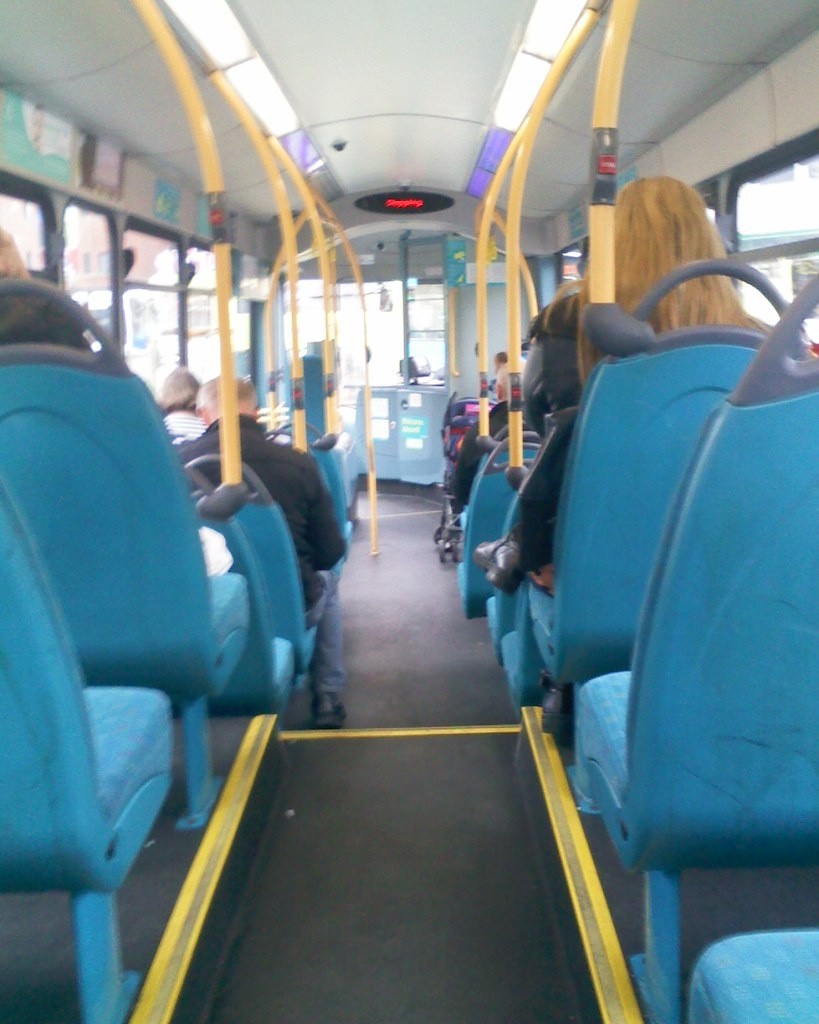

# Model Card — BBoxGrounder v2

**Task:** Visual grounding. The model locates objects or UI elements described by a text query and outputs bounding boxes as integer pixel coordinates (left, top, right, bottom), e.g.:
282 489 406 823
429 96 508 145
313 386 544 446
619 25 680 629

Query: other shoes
311 692 347 729
539 669 574 733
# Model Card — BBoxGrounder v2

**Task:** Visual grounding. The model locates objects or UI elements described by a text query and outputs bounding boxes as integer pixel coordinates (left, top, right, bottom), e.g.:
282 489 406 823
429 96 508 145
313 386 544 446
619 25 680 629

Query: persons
524 235 590 434
452 358 535 514
0 227 94 350
493 352 508 402
155 366 209 447
174 374 349 730
464 172 762 594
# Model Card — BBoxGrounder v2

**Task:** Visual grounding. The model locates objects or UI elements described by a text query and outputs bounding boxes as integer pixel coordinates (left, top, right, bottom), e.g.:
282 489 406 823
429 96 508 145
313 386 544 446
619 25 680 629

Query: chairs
182 452 318 673
278 422 354 564
177 465 296 716
0 477 174 1024
0 278 247 832
501 579 547 714
455 431 546 619
265 428 344 578
686 924 819 1024
295 356 328 437
527 259 809 811
460 419 540 541
583 267 819 1024
486 488 522 668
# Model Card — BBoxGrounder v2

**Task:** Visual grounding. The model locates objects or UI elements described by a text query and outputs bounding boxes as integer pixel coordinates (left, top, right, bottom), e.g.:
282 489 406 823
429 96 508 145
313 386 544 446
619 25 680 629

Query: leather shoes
473 533 527 594
